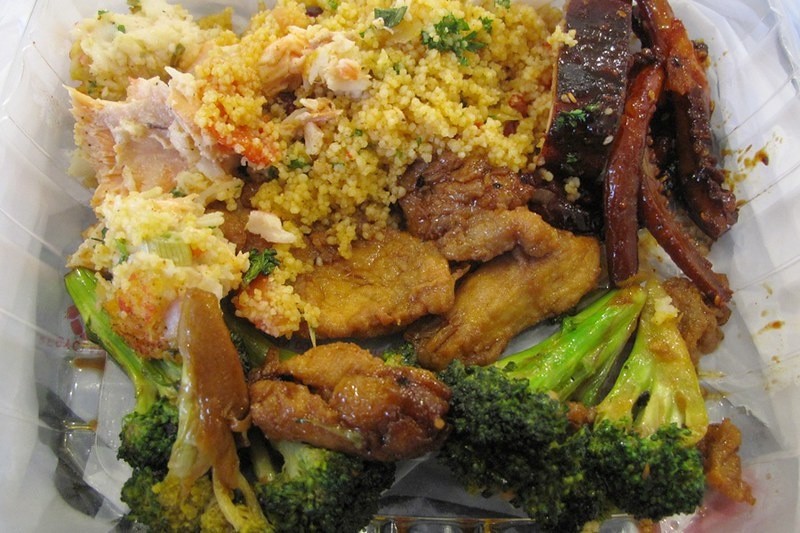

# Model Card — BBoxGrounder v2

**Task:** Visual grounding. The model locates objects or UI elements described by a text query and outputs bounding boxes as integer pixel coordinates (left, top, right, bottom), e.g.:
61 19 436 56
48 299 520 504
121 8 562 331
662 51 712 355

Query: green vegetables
374 6 599 128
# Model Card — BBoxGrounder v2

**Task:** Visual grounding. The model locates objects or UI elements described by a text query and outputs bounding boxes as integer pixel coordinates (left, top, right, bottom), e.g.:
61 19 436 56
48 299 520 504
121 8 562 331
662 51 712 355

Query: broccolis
65 249 708 533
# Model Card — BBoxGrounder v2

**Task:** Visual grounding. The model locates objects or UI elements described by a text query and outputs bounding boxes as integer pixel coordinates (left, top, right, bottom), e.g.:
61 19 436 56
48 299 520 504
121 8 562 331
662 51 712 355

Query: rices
68 0 579 342
